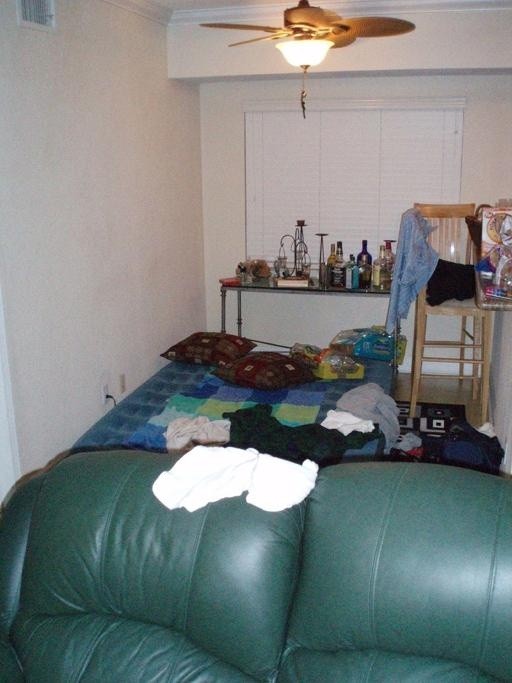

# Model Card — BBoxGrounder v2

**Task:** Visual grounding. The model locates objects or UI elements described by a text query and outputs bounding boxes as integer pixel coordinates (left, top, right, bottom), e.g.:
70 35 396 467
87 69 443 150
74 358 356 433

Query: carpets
375 401 466 462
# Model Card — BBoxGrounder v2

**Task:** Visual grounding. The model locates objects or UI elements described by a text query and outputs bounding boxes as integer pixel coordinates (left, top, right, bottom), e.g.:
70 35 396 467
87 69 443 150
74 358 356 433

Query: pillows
160 332 258 368
210 352 315 392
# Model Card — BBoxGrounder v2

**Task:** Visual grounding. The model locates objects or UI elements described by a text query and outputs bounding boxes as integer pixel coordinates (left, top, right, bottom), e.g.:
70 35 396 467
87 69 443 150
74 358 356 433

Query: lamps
274 39 335 120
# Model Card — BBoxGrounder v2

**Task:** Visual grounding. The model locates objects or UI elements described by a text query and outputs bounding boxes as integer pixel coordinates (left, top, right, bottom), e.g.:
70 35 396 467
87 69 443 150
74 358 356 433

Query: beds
71 352 395 465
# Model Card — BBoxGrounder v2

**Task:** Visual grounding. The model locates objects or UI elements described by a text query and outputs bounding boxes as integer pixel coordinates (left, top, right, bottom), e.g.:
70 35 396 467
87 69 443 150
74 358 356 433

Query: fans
198 1 415 48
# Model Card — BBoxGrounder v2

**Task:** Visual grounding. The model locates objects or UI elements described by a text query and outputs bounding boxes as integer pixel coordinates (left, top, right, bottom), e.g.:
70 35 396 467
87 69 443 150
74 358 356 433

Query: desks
219 276 416 374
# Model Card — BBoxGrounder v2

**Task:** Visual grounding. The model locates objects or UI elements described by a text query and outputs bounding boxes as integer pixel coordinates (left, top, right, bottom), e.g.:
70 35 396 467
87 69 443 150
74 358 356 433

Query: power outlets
101 384 109 405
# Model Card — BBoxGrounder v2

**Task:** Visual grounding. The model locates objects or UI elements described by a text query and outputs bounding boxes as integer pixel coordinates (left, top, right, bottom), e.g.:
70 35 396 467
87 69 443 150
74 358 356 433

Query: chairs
408 202 492 426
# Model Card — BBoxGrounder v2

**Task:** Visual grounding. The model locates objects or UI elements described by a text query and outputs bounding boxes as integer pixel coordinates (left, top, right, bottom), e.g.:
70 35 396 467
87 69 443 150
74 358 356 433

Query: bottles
343 254 353 268
346 256 359 289
331 241 346 287
359 254 372 288
378 257 394 292
385 240 395 264
357 240 372 266
327 243 336 281
372 245 385 289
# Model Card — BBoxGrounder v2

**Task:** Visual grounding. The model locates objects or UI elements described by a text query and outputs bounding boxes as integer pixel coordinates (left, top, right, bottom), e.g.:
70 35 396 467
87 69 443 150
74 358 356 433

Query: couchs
276 461 511 683
0 448 309 683
